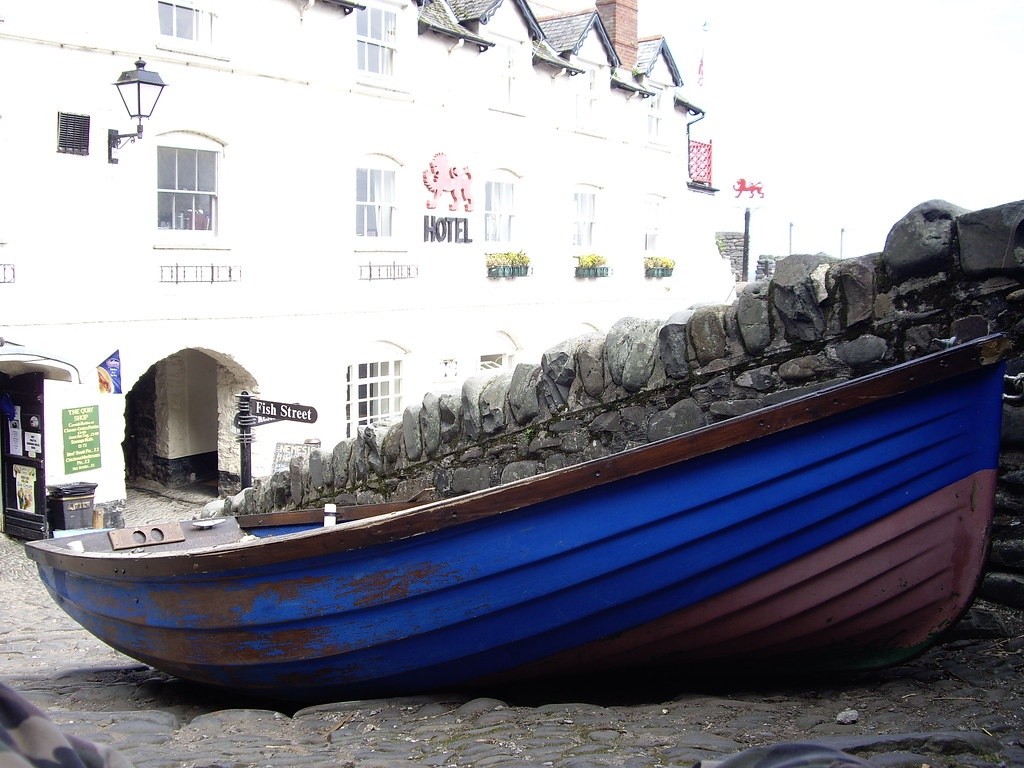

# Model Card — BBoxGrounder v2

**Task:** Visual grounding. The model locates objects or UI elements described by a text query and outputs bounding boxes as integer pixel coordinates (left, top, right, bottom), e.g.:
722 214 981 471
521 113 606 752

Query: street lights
840 228 845 257
789 222 793 255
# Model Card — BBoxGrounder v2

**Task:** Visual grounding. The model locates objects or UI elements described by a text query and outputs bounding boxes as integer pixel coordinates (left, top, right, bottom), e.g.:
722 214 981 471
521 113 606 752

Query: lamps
108 57 170 163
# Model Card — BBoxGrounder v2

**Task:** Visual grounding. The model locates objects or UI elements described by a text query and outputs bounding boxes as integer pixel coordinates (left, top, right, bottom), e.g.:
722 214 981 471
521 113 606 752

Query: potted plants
644 256 675 277
576 255 610 278
487 252 531 279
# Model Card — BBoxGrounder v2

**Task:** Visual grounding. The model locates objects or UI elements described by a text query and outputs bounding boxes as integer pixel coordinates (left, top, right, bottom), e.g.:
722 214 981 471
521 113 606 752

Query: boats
24 329 1013 716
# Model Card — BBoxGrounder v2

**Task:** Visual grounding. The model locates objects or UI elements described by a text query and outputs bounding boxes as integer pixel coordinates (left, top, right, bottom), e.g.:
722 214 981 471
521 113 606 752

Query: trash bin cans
47 481 98 528
